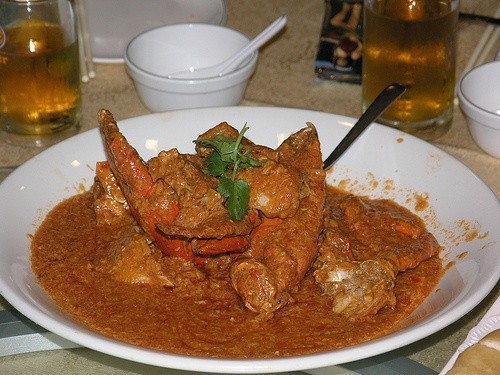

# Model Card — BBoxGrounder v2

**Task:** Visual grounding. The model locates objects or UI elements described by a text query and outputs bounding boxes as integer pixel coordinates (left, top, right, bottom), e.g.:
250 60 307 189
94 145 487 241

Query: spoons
167 16 288 78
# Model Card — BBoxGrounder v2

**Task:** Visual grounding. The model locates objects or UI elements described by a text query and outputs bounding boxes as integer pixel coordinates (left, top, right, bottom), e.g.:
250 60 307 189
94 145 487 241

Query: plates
94 0 227 64
0 106 500 374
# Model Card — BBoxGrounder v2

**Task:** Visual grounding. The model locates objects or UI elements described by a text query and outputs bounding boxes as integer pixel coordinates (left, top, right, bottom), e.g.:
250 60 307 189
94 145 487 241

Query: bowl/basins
457 60 500 159
124 22 258 113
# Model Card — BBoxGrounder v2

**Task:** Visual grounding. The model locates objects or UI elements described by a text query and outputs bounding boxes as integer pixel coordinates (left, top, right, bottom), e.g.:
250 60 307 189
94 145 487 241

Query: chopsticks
75 0 96 83
463 7 500 82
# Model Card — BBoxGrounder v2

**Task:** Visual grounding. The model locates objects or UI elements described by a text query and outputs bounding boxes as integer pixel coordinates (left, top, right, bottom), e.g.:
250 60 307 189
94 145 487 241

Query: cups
0 0 82 148
362 0 459 141
